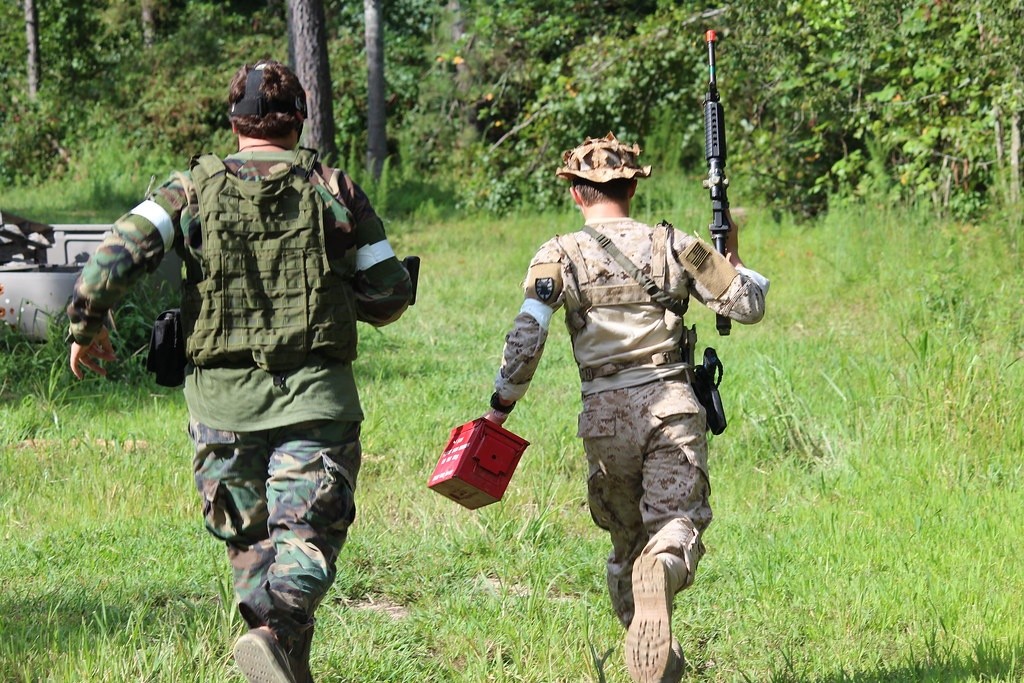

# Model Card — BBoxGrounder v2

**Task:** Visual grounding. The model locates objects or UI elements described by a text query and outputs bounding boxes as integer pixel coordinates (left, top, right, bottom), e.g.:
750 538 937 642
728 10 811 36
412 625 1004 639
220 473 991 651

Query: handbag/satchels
146 308 184 387
690 365 727 435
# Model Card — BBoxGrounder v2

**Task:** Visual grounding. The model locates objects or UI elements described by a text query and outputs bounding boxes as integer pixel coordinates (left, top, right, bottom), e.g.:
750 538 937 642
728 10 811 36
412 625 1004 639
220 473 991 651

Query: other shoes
625 553 684 683
233 629 314 683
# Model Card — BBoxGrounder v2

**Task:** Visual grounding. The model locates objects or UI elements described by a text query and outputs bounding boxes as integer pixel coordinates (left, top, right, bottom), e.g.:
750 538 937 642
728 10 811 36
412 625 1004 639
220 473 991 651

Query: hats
555 131 651 183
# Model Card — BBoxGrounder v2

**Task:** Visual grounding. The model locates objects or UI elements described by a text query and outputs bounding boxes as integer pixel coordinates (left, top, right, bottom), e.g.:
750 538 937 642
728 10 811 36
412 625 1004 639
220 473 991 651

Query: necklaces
239 144 289 152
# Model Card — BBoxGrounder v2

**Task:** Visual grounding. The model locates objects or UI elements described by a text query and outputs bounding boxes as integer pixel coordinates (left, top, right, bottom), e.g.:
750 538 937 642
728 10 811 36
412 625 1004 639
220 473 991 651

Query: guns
703 29 733 337
698 345 724 404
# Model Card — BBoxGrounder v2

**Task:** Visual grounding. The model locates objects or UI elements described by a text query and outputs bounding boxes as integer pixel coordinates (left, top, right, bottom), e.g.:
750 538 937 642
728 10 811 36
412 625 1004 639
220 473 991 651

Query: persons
65 60 420 683
484 130 770 683
